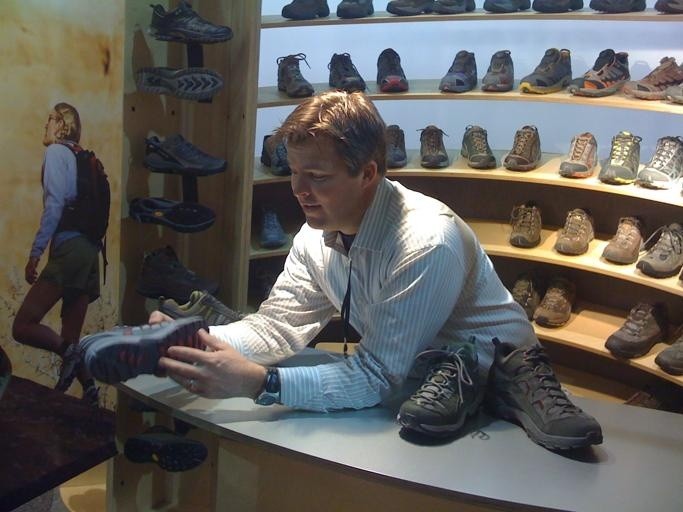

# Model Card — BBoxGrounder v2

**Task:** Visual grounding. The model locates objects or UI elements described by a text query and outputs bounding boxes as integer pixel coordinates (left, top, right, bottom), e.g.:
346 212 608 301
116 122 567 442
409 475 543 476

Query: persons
148 89 546 413
10 102 111 407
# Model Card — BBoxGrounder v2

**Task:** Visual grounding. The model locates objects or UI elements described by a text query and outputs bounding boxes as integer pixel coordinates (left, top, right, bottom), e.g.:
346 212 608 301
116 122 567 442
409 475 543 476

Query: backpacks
57 141 110 237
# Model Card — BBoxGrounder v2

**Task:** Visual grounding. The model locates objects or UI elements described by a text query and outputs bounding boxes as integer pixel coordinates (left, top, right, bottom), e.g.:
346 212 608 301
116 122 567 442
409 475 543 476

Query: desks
0 375 118 512
112 347 683 512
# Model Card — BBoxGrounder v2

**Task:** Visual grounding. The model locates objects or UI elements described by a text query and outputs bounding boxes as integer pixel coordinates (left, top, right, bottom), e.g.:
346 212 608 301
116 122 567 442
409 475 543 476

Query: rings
188 380 197 392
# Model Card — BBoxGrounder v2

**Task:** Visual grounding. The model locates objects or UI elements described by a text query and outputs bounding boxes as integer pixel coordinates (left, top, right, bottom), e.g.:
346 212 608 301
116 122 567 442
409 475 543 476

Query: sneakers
55 343 98 403
486 337 603 450
143 134 228 178
397 336 487 437
276 48 408 99
260 134 291 176
135 243 247 325
77 316 210 384
258 201 286 248
136 66 224 99
505 125 540 172
128 196 216 232
384 123 450 169
558 132 683 188
605 300 683 411
460 126 497 168
124 425 207 472
510 200 683 281
149 3 234 44
439 49 683 105
510 271 576 328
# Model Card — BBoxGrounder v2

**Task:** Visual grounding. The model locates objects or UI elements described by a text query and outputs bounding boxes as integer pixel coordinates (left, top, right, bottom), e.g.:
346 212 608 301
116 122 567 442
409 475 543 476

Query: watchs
254 367 281 406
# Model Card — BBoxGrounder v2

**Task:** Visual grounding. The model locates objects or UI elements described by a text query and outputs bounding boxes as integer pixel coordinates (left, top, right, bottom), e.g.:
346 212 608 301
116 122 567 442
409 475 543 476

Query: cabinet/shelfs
119 0 683 415
